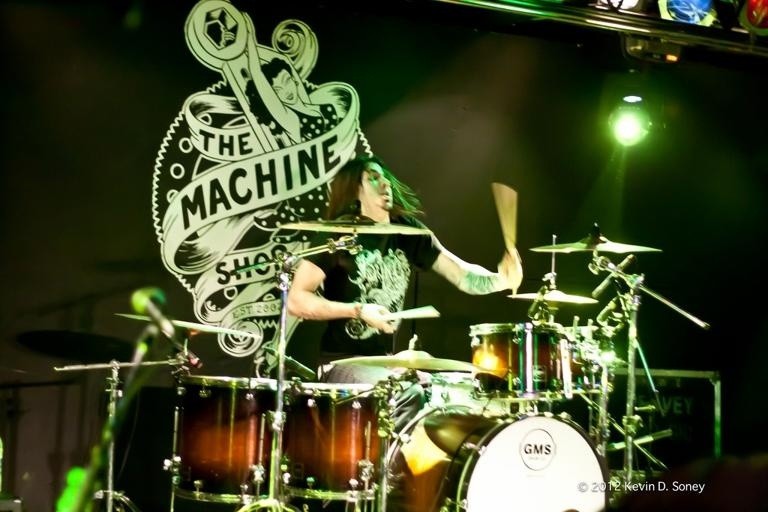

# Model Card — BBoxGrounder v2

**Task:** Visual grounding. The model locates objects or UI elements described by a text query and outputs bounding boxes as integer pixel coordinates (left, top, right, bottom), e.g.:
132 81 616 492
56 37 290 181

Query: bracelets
355 297 363 320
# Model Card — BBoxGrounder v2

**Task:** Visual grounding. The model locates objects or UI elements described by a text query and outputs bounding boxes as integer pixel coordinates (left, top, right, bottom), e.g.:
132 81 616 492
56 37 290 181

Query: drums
281 383 387 503
175 373 289 508
468 323 562 386
384 401 608 512
560 325 606 378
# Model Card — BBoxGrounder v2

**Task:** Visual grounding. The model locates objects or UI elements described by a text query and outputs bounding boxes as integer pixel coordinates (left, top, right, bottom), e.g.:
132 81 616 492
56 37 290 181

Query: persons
285 158 523 432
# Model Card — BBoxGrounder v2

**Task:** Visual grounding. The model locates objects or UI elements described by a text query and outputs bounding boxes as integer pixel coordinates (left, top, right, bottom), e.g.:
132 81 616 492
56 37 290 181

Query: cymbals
507 291 600 306
123 311 261 339
529 238 664 255
280 215 431 240
329 350 473 373
